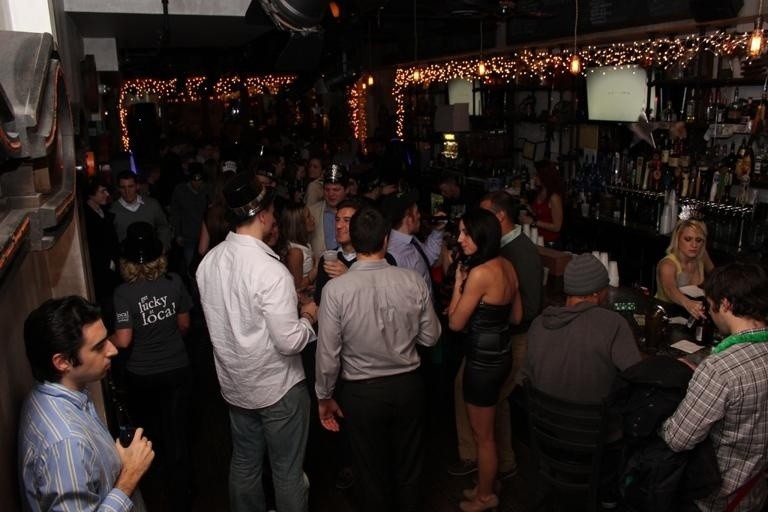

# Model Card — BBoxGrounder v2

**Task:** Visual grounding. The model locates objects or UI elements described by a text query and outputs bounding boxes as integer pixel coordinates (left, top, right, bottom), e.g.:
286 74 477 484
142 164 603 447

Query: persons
651 218 716 323
662 261 766 511
83 142 567 510
15 296 157 511
510 250 662 511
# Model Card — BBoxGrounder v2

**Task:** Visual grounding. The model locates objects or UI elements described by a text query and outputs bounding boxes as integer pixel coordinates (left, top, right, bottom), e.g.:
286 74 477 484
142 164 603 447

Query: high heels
459 495 498 512
463 483 498 500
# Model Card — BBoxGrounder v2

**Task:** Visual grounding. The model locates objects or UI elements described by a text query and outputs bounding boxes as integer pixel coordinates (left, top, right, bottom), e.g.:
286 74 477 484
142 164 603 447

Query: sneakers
496 458 516 481
447 458 479 476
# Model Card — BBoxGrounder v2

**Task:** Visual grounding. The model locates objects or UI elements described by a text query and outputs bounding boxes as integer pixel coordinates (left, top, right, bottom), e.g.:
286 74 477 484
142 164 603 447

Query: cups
580 202 588 217
322 250 338 264
514 222 619 290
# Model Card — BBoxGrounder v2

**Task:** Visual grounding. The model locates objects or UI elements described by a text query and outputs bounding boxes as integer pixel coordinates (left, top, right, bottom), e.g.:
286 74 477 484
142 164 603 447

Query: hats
319 163 348 186
221 170 275 223
114 219 164 265
562 254 610 295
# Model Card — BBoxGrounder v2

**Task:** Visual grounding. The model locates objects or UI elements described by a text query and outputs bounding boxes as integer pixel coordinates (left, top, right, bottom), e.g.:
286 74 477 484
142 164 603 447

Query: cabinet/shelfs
471 48 764 215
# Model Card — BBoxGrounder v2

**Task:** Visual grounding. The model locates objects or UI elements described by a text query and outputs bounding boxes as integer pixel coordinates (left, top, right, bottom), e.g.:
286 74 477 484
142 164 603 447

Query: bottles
113 399 136 448
609 84 768 208
492 168 529 191
583 154 597 175
695 307 714 346
